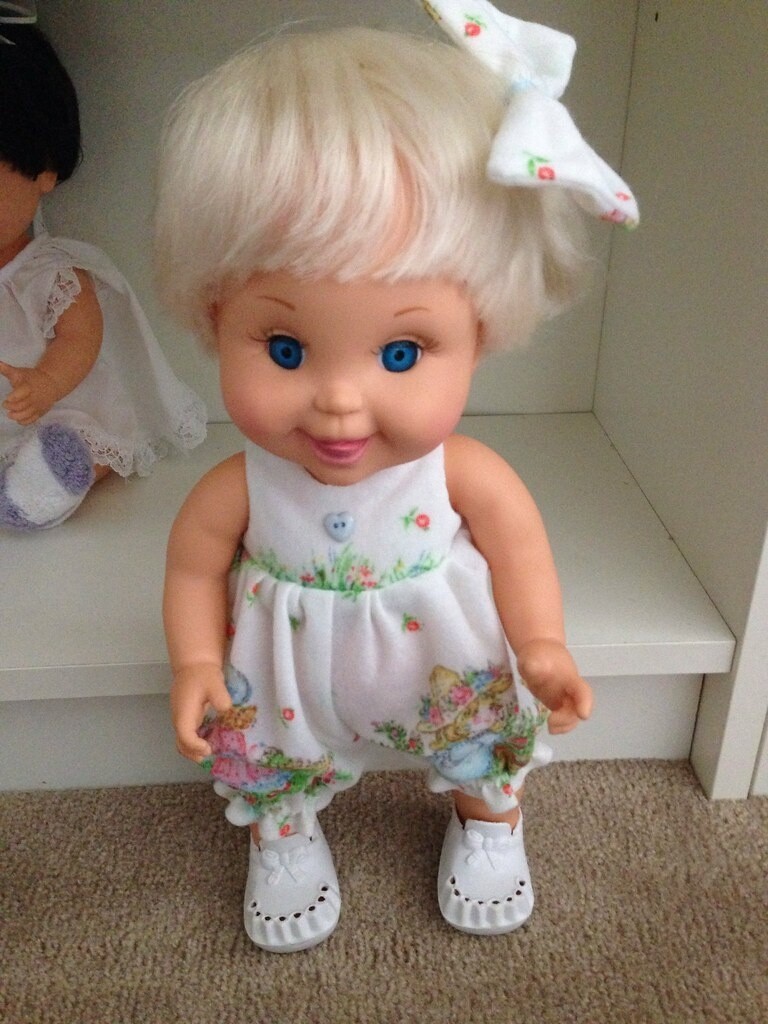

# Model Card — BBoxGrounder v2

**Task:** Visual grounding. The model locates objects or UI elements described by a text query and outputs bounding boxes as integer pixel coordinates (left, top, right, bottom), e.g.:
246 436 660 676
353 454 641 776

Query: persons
158 3 645 955
0 0 211 532
